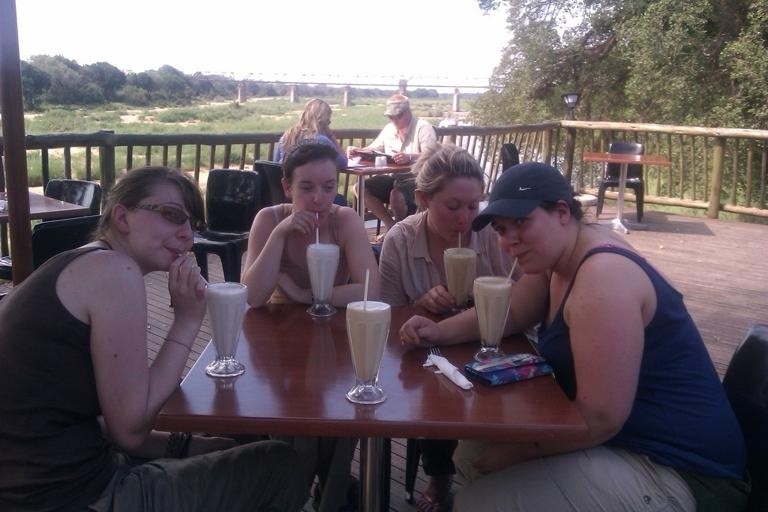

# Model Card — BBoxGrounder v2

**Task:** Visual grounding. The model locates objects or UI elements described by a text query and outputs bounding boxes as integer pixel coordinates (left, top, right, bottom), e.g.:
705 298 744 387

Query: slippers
415 490 448 512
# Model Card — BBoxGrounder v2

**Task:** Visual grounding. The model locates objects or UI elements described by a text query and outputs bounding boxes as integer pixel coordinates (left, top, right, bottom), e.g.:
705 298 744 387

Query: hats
470 162 576 233
383 94 410 116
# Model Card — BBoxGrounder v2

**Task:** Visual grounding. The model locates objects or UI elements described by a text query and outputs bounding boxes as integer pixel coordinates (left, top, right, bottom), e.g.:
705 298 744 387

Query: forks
430 345 442 360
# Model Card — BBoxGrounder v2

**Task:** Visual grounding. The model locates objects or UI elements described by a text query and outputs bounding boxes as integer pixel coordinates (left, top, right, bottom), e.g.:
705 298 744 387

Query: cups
375 156 387 168
306 244 340 317
204 281 248 378
473 276 513 363
345 301 392 404
443 248 476 312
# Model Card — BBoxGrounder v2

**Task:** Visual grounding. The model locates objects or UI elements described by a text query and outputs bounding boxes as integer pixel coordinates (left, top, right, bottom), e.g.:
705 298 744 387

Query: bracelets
327 131 335 138
166 431 192 459
408 156 412 163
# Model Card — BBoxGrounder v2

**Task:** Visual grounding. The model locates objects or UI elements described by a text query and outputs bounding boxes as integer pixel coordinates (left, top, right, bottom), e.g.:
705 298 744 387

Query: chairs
43 179 102 223
251 158 289 210
1 213 105 280
166 167 269 307
501 142 519 173
720 323 768 510
595 131 647 223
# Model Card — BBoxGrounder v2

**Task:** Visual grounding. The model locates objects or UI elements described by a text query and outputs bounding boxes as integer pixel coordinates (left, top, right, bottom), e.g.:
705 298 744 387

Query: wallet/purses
463 353 553 386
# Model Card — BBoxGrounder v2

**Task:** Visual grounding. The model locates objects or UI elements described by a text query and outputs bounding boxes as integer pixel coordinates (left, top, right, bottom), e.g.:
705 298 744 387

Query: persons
0 165 310 511
379 143 522 512
241 140 382 512
274 99 349 207
346 94 438 245
398 161 753 512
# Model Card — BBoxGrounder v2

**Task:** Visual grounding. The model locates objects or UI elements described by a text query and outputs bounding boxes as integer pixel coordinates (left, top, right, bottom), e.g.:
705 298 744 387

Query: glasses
128 202 209 237
